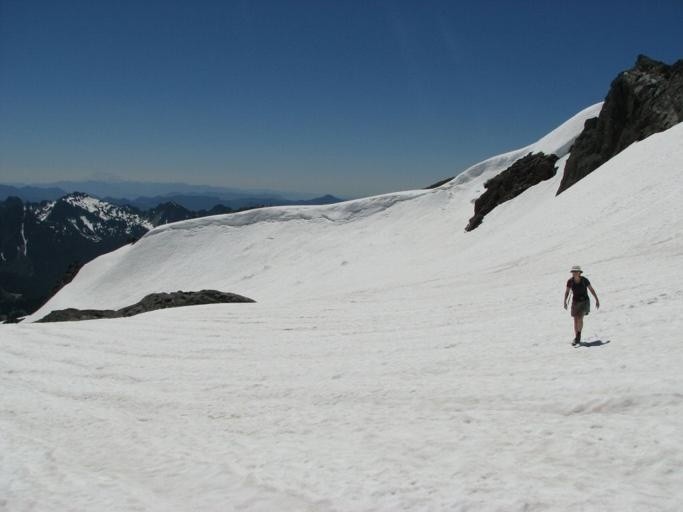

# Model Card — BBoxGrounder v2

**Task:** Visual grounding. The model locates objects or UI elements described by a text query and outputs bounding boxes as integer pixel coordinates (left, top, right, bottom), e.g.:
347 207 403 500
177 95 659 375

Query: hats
570 266 583 273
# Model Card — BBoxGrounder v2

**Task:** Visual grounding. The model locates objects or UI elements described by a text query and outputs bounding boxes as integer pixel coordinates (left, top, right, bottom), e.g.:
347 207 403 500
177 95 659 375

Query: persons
562 265 599 346
